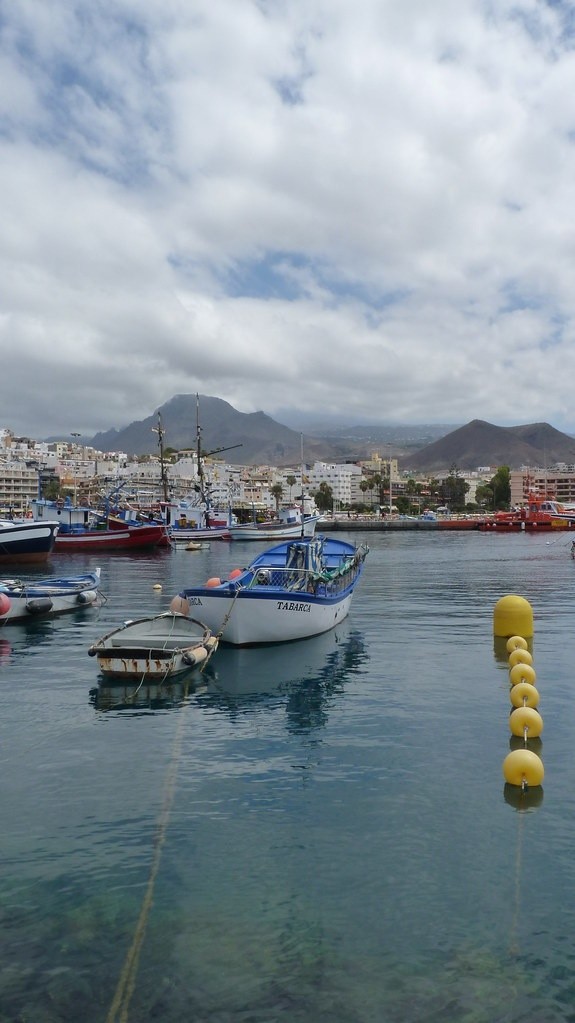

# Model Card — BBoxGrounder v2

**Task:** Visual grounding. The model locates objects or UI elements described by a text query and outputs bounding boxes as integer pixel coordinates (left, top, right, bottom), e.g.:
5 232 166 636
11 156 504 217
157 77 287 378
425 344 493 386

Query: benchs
112 635 204 649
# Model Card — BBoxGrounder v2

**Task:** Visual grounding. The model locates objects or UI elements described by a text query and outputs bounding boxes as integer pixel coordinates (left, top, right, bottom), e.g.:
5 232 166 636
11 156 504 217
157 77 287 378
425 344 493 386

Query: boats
1 599 101 661
87 610 211 679
88 669 209 717
0 516 60 565
0 568 101 624
181 434 371 648
476 498 575 531
11 470 170 554
90 481 323 544
